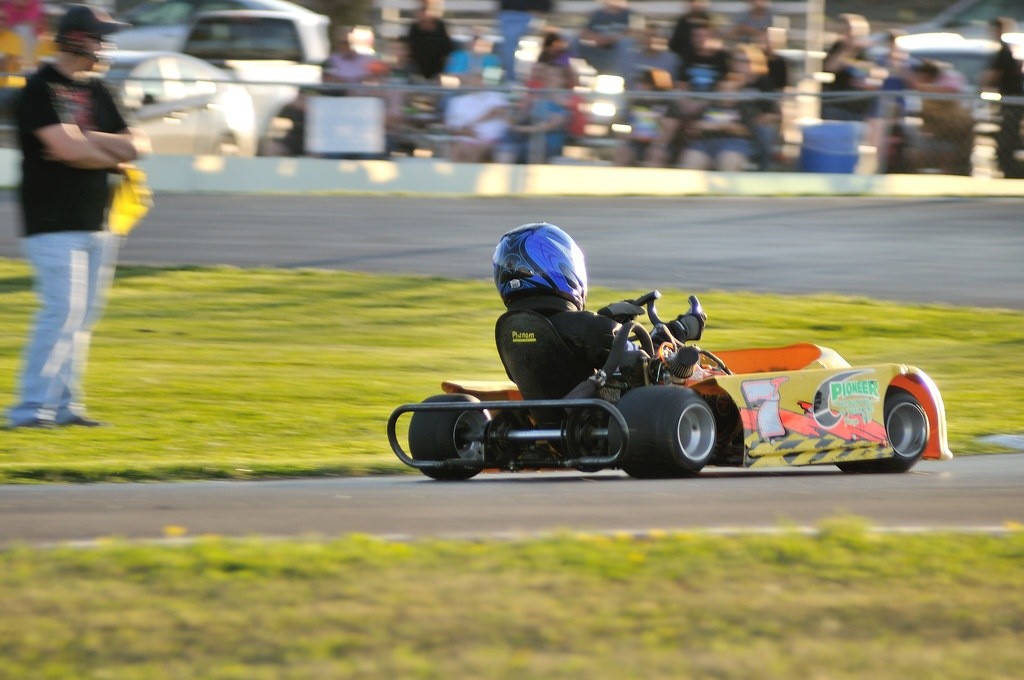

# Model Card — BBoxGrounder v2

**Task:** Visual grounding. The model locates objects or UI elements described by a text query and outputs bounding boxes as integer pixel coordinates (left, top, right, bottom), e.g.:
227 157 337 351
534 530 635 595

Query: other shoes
72 415 107 428
30 419 56 430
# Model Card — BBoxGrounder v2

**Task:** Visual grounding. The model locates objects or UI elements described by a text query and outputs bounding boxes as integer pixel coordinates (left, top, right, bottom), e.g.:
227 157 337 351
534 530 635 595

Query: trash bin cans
799 121 862 174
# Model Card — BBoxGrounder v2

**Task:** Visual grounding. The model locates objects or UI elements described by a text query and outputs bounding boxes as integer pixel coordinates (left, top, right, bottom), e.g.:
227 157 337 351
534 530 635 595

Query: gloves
667 313 707 342
599 299 646 322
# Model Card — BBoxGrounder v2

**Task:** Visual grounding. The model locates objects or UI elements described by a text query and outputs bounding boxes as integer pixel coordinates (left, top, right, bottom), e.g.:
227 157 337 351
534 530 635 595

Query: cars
99 1 331 67
0 50 258 160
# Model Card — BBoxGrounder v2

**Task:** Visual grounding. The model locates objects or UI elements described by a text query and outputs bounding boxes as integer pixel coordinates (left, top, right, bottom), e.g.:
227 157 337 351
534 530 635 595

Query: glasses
85 33 114 42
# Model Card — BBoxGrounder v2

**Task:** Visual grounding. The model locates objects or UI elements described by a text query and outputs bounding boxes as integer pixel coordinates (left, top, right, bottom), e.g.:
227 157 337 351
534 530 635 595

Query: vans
181 5 321 158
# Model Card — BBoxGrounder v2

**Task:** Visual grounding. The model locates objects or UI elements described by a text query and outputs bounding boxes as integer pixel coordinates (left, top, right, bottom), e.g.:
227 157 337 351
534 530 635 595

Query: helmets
493 222 587 310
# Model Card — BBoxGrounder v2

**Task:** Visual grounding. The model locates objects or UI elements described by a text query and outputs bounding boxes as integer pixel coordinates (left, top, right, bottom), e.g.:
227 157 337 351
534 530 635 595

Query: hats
58 4 132 34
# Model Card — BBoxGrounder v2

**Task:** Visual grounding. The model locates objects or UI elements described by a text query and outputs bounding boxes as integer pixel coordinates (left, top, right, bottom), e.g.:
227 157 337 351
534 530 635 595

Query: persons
492 220 704 372
272 0 1024 179
0 7 152 428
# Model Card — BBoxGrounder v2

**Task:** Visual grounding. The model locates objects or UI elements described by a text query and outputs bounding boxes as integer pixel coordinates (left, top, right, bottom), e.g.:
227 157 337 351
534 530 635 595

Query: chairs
495 308 631 425
